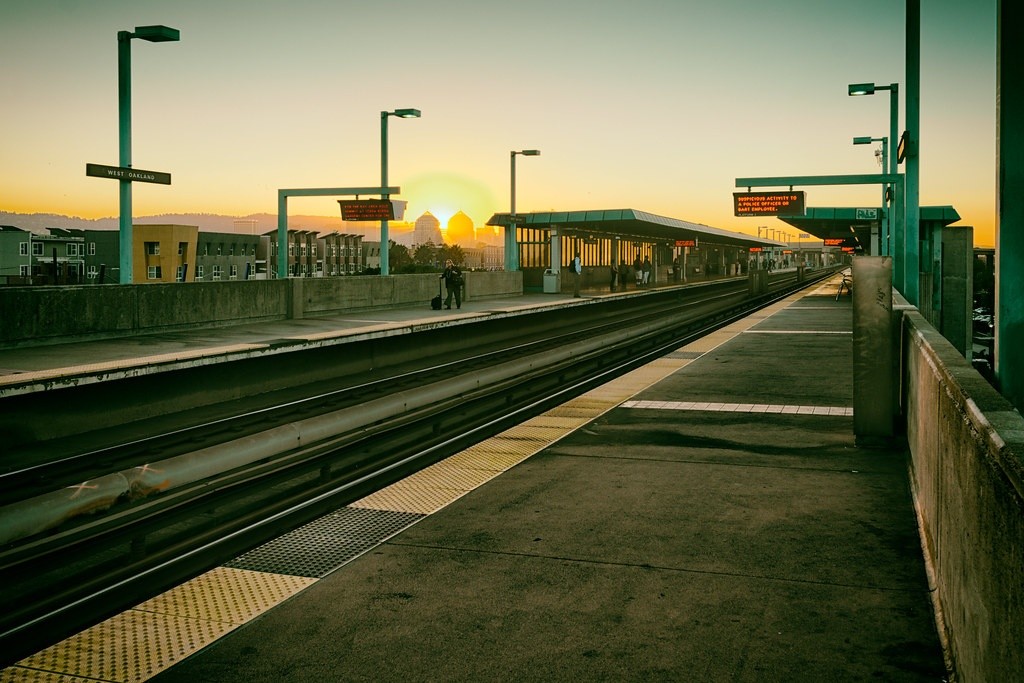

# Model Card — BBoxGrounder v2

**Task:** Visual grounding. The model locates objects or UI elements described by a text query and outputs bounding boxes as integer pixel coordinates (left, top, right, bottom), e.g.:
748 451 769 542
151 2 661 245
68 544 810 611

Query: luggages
431 278 442 310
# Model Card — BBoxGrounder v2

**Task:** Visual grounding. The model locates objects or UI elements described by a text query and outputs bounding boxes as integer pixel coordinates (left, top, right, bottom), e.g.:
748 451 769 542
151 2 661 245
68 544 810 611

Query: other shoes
574 295 580 298
457 306 460 309
447 306 451 309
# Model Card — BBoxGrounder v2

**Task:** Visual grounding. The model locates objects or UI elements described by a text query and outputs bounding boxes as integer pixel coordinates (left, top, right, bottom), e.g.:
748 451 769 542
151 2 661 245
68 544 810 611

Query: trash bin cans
542 268 563 294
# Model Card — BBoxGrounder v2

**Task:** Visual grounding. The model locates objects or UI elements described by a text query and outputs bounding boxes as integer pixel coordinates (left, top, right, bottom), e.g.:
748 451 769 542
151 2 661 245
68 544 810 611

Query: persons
439 260 462 310
644 256 651 282
618 259 629 291
734 255 788 276
641 260 650 285
573 253 582 297
703 260 710 280
633 255 643 287
671 258 678 280
610 258 618 292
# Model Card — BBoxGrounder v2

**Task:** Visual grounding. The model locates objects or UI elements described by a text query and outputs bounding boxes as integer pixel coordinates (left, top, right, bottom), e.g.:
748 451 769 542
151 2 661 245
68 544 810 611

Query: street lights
853 135 888 257
848 81 899 288
510 149 540 270
380 108 421 274
117 23 180 285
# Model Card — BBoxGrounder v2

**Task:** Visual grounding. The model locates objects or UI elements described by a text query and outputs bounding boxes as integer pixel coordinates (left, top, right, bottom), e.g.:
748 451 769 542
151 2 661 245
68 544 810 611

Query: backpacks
569 258 577 272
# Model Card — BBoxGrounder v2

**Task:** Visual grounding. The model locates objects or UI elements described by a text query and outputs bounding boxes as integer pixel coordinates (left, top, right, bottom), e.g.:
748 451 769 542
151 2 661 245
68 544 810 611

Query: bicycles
835 271 852 302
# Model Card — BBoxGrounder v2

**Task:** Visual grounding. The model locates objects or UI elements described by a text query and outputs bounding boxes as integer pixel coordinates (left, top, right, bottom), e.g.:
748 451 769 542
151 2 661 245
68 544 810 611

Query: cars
972 300 996 379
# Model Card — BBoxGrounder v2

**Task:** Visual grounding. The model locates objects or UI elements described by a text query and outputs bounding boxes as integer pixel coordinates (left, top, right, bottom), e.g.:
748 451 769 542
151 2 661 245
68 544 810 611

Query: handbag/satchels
455 277 464 285
